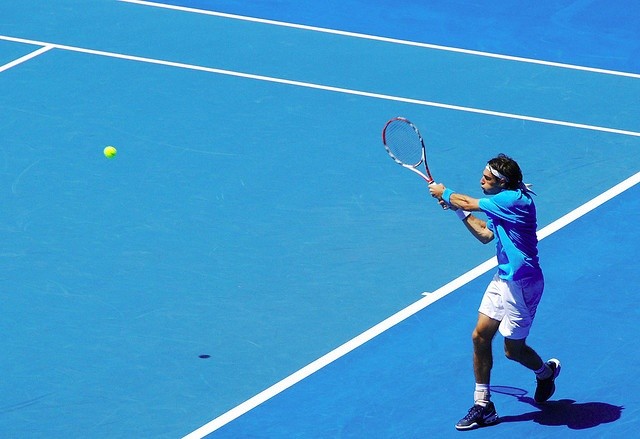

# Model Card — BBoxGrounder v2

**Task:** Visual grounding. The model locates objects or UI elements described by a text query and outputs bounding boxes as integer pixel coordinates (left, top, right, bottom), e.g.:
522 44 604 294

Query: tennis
103 146 118 160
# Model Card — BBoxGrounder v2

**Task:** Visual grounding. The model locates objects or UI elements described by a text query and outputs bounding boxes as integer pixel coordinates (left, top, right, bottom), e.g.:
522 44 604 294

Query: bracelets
455 208 472 220
441 188 454 204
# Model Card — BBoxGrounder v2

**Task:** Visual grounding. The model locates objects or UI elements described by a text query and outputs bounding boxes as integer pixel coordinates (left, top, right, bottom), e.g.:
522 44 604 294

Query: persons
428 153 561 430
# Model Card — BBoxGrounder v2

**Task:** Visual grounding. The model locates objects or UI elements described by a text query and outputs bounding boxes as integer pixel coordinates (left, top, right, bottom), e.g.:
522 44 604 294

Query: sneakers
533 357 561 404
454 402 499 430
